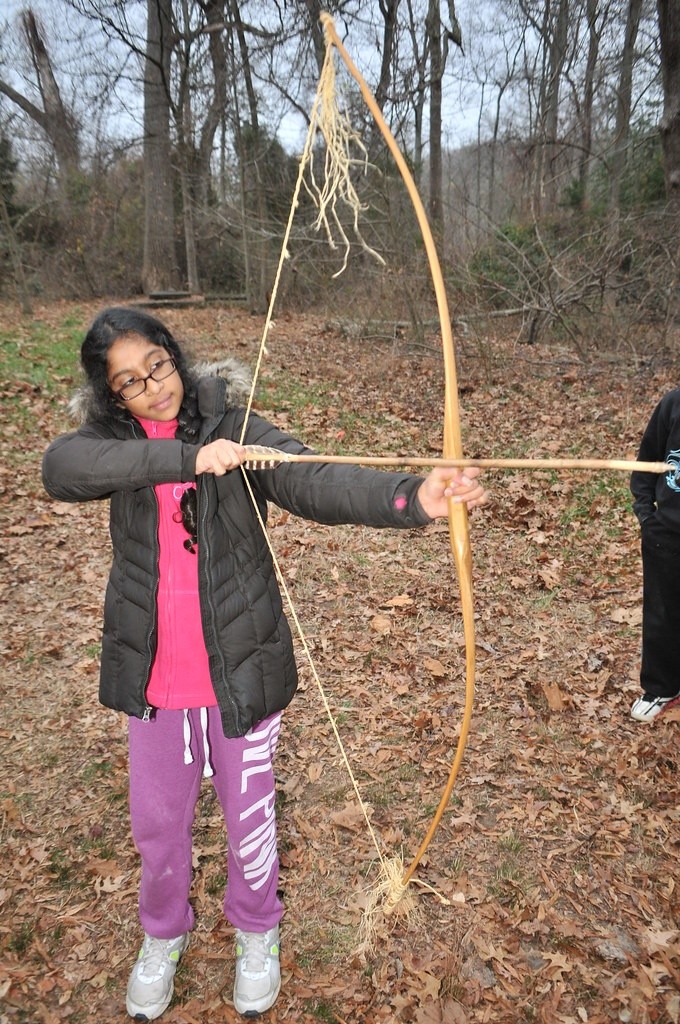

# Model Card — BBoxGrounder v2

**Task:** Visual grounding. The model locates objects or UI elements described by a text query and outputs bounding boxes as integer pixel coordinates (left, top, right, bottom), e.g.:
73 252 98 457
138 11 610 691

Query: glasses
109 344 178 402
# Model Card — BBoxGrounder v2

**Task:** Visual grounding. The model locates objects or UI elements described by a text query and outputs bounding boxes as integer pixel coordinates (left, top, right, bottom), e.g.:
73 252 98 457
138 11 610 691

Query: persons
41 307 490 1020
629 390 680 722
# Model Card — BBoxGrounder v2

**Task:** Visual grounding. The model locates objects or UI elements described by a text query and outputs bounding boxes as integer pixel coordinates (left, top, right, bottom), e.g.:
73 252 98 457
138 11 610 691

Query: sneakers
126 931 190 1019
630 691 680 720
232 922 281 1016
